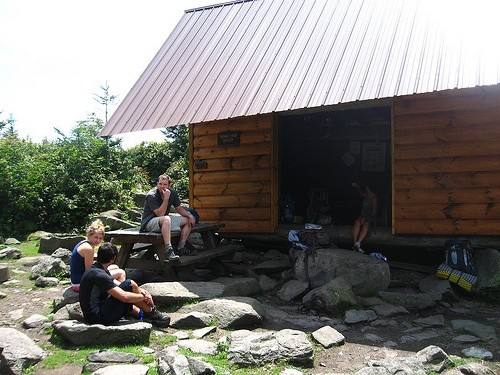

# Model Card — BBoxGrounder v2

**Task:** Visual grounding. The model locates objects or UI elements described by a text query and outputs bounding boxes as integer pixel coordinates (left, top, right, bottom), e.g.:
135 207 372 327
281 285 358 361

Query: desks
106 223 229 281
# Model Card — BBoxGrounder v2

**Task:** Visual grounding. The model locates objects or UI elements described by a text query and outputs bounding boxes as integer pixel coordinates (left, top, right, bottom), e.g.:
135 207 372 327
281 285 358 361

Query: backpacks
441 239 478 295
299 227 332 250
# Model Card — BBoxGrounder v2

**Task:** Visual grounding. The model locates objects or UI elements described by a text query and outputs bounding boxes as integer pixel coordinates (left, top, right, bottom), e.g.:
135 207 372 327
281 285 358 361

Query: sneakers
151 322 169 328
165 247 180 260
175 247 191 256
143 306 169 324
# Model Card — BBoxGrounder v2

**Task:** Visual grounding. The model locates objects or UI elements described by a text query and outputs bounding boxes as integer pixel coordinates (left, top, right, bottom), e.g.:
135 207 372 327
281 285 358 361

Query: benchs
93 243 153 257
162 243 246 267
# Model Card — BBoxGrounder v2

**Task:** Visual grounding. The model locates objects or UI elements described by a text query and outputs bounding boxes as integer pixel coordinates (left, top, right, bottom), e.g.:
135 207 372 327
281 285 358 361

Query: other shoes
352 243 364 254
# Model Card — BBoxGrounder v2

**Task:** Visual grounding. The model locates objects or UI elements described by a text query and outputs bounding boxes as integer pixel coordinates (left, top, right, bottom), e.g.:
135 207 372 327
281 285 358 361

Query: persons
70 219 126 293
139 173 196 261
79 241 170 329
352 182 378 253
